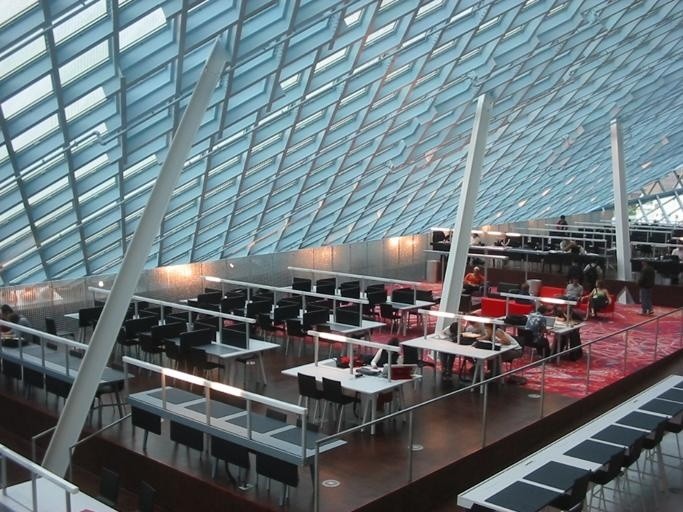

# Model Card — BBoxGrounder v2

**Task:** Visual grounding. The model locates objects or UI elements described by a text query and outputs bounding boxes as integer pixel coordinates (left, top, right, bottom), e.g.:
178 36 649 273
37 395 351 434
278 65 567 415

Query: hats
474 267 480 271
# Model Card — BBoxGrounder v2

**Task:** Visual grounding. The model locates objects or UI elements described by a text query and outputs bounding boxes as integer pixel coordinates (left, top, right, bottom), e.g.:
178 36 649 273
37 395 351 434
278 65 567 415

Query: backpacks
335 354 367 368
474 342 500 351
453 335 476 345
585 263 599 282
504 314 528 325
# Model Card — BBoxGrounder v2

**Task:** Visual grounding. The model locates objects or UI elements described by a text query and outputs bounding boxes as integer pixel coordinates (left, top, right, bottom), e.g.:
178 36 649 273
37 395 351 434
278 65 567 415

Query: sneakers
639 310 655 316
582 313 595 321
441 372 453 381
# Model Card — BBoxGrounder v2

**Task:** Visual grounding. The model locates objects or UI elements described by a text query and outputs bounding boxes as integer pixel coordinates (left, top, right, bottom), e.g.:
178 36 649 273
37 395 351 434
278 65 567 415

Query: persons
438 319 466 382
369 337 405 417
443 231 453 242
523 304 553 364
462 320 490 374
483 323 523 384
461 215 682 319
0 313 33 344
0 304 13 332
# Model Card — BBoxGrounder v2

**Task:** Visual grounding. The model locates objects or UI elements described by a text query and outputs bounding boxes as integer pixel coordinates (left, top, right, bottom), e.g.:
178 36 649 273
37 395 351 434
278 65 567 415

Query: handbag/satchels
590 297 608 308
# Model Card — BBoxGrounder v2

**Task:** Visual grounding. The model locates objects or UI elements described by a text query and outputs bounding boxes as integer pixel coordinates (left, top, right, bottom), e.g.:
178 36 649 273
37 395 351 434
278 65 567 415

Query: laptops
380 364 419 379
545 316 557 329
